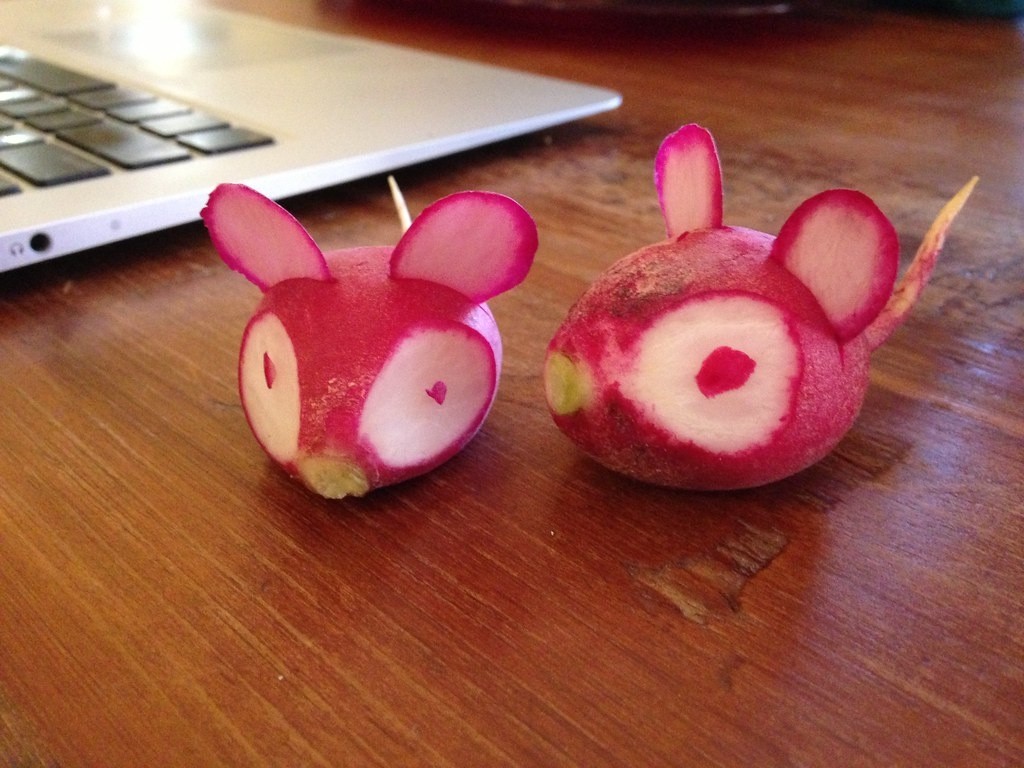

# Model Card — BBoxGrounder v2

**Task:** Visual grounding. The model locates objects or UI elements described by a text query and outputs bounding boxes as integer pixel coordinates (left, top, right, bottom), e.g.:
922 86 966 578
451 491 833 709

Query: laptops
0 0 623 269
